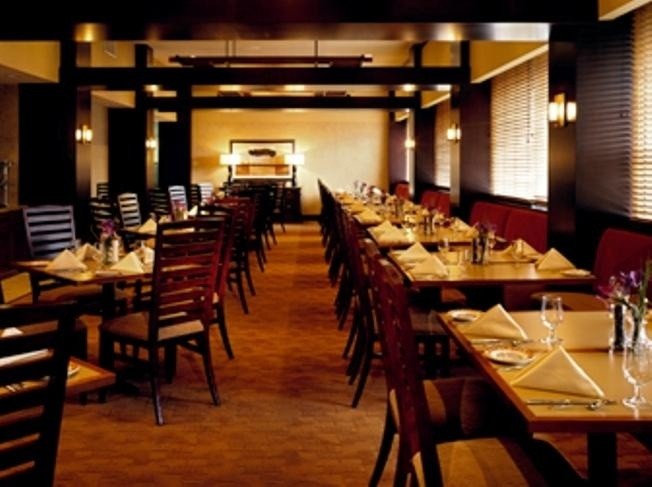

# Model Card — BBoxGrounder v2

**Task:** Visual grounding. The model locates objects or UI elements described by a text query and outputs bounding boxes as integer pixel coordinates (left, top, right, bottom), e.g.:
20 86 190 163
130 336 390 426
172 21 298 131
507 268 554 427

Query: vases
620 320 651 411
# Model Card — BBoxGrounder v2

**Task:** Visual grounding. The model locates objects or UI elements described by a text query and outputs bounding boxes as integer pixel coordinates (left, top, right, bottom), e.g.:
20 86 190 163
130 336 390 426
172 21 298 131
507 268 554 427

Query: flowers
597 258 652 325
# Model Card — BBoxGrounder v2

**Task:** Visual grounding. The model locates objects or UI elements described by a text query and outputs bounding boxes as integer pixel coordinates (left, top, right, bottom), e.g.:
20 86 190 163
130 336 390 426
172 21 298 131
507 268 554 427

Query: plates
482 343 536 365
527 254 545 260
447 308 485 321
559 268 590 277
66 361 80 377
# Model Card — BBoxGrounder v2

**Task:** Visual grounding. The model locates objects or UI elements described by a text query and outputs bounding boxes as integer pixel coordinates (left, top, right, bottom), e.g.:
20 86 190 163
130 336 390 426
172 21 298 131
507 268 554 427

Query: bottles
111 236 118 262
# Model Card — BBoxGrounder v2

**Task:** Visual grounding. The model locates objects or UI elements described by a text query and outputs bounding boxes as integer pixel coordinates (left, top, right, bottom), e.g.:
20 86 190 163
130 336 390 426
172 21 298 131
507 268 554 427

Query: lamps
284 153 306 186
75 122 95 145
545 93 577 129
445 121 462 143
219 153 241 183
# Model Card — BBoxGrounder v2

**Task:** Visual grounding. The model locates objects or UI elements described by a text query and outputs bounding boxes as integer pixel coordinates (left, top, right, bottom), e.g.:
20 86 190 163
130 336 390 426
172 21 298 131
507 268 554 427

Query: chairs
0 204 74 325
22 205 74 300
1 299 80 486
315 176 598 410
367 271 532 487
95 216 227 426
89 177 287 360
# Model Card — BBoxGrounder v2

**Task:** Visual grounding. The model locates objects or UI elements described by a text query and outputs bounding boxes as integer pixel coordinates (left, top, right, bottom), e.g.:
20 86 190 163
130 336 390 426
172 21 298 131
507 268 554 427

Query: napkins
463 301 530 344
108 251 147 275
137 245 156 271
513 346 605 399
47 249 88 274
77 242 104 263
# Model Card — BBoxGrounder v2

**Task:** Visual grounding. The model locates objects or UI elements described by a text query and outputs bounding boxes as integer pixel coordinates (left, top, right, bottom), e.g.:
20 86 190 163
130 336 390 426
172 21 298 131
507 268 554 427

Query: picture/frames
230 139 296 167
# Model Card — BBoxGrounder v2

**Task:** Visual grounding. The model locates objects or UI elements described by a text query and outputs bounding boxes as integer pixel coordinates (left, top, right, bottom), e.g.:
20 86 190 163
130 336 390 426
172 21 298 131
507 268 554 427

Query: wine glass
511 239 524 267
618 339 652 410
132 239 147 268
487 230 498 257
540 295 565 345
65 237 107 273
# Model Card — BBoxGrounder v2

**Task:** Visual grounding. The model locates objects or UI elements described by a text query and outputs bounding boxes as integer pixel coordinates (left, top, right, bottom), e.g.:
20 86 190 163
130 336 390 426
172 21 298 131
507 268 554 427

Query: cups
437 237 450 261
455 248 474 277
